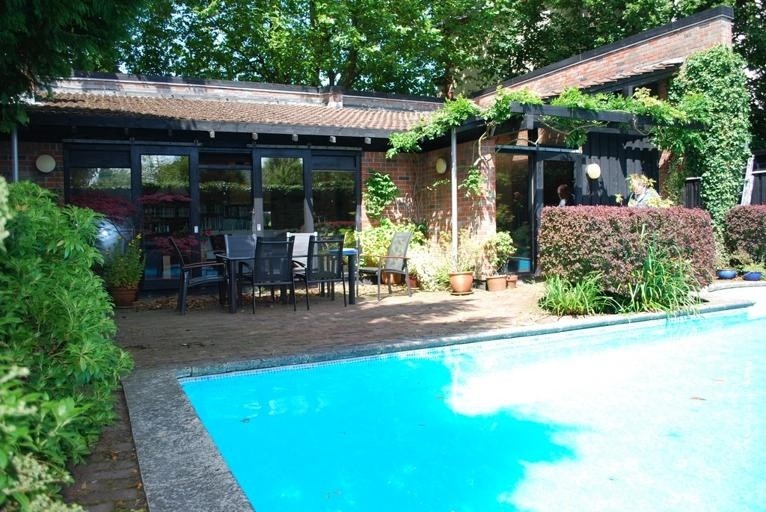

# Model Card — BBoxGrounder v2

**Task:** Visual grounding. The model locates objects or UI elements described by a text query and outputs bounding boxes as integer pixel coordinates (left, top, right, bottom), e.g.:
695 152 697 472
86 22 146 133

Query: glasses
365 137 372 144
252 132 258 140
210 129 215 138
436 157 447 174
585 163 602 179
35 154 56 173
292 134 299 141
330 135 336 142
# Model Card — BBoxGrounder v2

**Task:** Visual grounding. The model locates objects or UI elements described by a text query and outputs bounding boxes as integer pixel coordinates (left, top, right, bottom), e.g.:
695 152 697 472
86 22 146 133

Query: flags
716 269 737 279
110 288 139 308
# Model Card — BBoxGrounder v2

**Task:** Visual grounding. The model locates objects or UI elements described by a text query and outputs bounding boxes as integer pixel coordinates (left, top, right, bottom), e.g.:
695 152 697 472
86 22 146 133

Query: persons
625 173 662 209
557 184 576 207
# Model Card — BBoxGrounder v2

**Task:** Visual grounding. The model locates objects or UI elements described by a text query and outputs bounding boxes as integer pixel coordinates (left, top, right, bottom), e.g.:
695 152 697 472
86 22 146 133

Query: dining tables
405 230 518 295
741 261 765 280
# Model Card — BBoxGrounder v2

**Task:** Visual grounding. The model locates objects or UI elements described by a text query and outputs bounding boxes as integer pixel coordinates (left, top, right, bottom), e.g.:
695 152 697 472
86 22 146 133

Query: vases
101 231 146 289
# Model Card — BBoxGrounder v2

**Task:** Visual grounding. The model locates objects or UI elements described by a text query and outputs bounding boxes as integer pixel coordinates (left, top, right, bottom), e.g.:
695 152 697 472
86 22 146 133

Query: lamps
167 232 412 315
